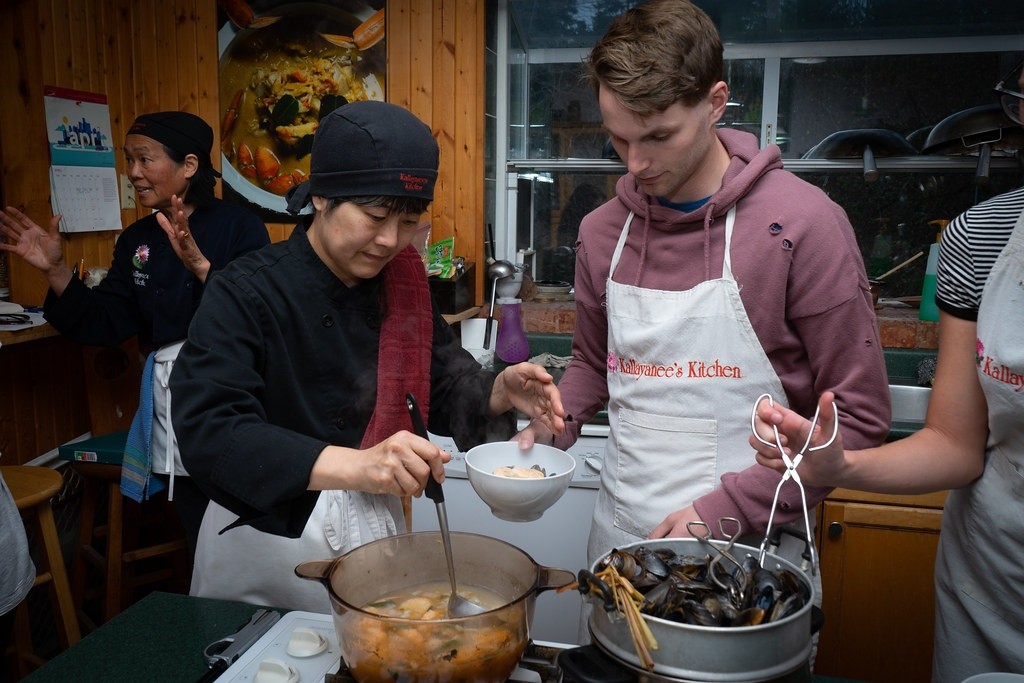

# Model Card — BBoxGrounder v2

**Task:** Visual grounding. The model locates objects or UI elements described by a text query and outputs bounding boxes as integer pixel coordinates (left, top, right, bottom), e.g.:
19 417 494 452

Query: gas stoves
211 610 578 683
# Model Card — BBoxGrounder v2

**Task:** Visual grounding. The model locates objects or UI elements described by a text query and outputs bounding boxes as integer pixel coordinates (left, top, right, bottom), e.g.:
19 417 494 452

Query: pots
557 537 824 682
295 531 576 683
799 103 1022 179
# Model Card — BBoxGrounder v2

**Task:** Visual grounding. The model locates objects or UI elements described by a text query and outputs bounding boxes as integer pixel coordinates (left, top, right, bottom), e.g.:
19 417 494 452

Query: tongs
685 515 750 609
751 393 839 575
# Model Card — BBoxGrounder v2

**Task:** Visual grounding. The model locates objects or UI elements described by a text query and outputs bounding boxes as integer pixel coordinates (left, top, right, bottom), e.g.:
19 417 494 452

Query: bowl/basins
495 272 523 301
219 4 387 219
464 442 576 522
867 277 884 307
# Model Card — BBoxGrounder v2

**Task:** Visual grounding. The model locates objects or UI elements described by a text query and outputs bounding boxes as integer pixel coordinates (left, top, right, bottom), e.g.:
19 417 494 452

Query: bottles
918 220 955 323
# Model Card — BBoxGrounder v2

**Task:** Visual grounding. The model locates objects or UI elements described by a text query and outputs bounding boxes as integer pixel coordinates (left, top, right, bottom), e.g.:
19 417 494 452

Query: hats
126 111 215 160
309 100 439 199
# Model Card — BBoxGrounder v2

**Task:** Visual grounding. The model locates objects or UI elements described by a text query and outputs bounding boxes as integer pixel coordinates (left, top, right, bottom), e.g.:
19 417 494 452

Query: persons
0 110 277 582
0 476 36 620
167 101 573 616
743 60 1024 683
503 0 897 646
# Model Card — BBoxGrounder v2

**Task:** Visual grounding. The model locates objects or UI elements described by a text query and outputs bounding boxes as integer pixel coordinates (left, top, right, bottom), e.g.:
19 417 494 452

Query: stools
66 463 191 623
0 465 82 683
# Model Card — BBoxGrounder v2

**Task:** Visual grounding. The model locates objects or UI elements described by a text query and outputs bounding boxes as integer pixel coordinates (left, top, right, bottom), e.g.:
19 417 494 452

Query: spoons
482 260 514 351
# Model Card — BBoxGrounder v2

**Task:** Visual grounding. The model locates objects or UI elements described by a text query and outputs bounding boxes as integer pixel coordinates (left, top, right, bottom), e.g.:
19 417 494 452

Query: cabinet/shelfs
810 487 949 682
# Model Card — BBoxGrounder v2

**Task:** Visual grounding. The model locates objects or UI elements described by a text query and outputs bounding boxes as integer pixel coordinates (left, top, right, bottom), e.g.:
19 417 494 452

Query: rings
183 233 190 238
